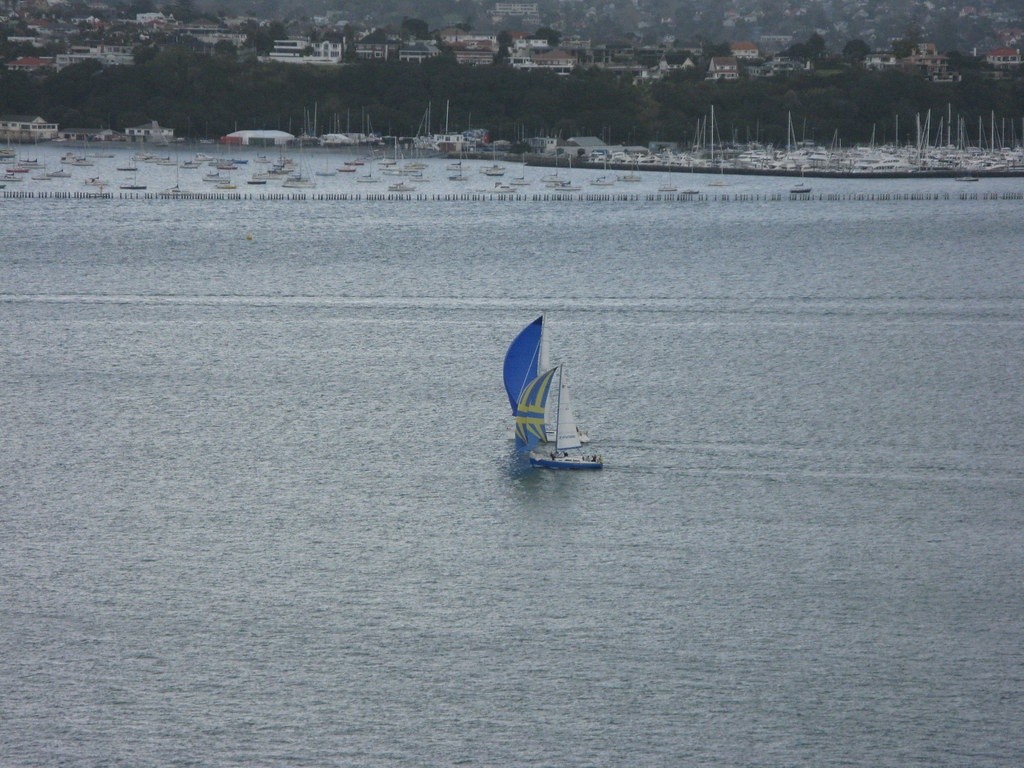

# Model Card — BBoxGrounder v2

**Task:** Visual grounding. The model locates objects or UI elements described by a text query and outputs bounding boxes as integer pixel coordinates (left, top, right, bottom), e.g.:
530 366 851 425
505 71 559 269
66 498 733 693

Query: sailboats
502 314 591 445
285 101 490 152
0 102 431 191
485 140 506 176
554 146 583 191
590 148 615 186
615 151 642 182
587 102 1024 177
509 153 532 186
516 361 605 471
658 155 678 191
446 131 470 170
544 181 571 186
541 142 567 183
450 150 470 182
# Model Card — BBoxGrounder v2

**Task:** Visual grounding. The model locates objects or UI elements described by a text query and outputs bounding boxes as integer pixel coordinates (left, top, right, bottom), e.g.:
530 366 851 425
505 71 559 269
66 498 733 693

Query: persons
586 454 601 463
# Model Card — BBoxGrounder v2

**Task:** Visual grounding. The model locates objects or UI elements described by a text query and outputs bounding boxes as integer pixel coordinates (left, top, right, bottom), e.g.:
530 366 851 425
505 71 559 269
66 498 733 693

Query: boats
954 177 979 181
789 182 812 193
487 185 517 194
682 189 700 194
709 179 732 186
